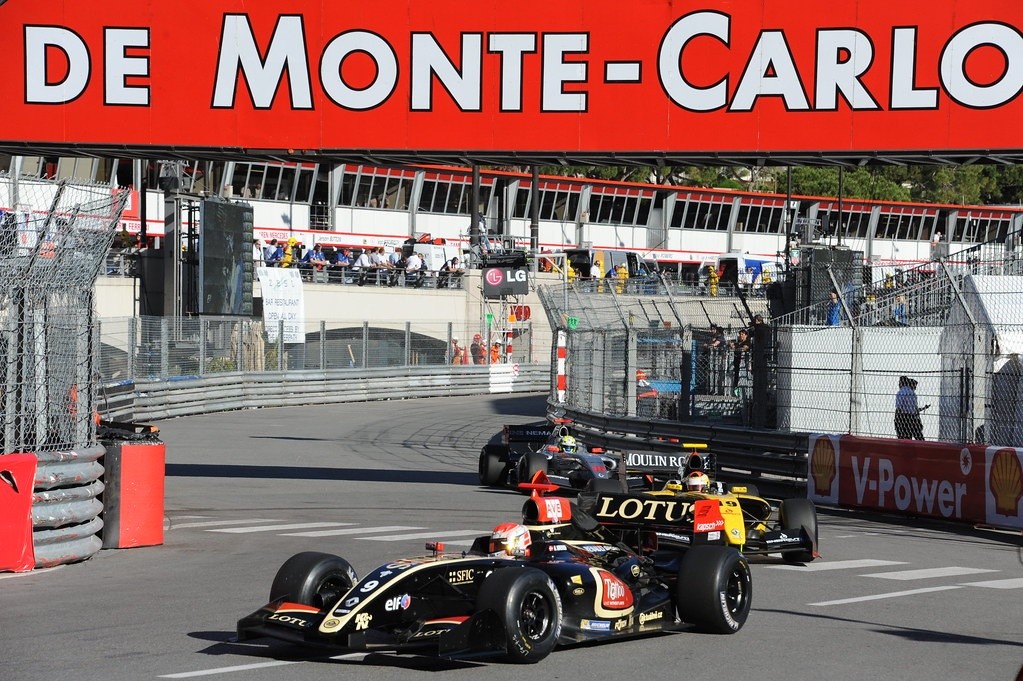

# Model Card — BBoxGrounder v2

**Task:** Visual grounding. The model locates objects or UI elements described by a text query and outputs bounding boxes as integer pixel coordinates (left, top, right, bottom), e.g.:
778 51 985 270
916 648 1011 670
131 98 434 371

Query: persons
550 258 575 290
741 266 753 294
452 334 501 364
590 259 674 295
703 313 771 384
559 435 576 454
934 230 941 236
893 376 932 442
708 266 719 297
685 471 710 493
825 270 934 328
251 237 458 288
488 522 532 556
240 183 285 201
967 255 980 274
876 232 889 238
134 233 148 253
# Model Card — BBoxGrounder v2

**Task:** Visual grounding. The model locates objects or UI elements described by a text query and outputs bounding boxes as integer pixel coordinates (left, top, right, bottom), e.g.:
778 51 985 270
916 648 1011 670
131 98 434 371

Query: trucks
563 249 651 280
717 254 786 294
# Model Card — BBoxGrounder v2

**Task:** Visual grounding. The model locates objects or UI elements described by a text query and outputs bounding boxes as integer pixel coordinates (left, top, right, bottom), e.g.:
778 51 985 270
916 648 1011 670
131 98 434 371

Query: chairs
470 530 549 562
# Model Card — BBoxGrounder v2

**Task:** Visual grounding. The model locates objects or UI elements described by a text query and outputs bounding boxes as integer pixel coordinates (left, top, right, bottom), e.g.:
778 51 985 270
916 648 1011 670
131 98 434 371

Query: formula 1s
234 419 825 667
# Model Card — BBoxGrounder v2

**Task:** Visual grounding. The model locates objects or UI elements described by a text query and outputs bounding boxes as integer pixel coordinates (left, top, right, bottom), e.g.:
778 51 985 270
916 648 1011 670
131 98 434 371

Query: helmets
559 436 576 453
765 270 768 273
686 471 710 493
886 274 891 277
621 263 626 267
708 266 714 272
494 340 502 346
636 370 647 379
287 238 297 246
474 334 481 341
489 523 532 557
